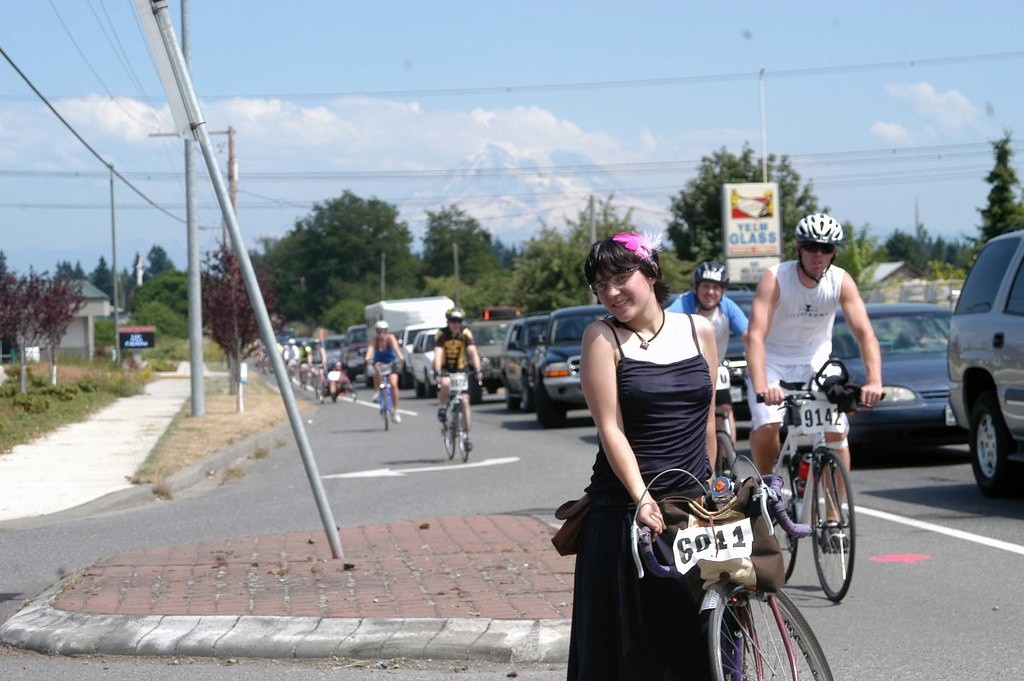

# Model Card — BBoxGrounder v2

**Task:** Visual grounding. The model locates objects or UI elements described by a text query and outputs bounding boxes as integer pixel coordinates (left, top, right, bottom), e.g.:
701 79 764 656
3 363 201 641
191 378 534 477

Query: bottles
796 452 812 498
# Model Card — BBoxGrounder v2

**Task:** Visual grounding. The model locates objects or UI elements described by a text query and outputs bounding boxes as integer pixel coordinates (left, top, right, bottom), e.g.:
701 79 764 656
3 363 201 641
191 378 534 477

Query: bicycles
371 359 399 431
714 362 745 480
756 358 886 602
630 456 834 681
257 357 325 401
433 359 490 462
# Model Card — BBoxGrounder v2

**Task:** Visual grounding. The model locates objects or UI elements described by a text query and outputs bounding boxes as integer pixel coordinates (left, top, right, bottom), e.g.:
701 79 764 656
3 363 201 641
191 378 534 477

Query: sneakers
820 519 851 552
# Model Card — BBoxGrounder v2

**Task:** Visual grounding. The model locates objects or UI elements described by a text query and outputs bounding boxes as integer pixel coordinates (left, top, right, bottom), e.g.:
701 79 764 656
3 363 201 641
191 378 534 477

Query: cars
831 303 969 469
665 291 753 420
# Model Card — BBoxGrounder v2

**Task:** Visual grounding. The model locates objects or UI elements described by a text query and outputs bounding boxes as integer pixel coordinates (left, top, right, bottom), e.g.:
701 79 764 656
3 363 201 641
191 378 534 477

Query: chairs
831 336 848 359
561 321 580 337
843 334 860 357
894 331 918 350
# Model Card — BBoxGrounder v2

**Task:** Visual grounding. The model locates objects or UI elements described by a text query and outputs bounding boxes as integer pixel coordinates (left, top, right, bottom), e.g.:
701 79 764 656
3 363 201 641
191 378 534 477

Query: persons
743 213 883 550
432 307 483 451
566 230 730 681
364 321 405 422
663 260 750 493
255 338 350 397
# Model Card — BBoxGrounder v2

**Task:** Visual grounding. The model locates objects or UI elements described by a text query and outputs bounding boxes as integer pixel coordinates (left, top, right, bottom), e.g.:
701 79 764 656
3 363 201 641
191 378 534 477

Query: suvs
275 297 613 428
946 230 1024 499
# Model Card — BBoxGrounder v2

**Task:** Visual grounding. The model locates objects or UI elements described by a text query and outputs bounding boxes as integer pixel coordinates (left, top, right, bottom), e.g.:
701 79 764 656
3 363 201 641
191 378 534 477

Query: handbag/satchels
658 477 784 592
551 493 596 558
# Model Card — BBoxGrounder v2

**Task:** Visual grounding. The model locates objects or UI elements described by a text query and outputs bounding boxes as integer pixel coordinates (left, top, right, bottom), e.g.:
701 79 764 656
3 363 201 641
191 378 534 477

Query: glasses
587 263 644 292
451 317 464 324
798 240 837 255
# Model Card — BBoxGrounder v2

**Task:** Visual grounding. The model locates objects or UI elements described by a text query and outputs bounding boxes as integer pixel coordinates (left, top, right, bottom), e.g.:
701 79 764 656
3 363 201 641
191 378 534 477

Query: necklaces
630 310 666 349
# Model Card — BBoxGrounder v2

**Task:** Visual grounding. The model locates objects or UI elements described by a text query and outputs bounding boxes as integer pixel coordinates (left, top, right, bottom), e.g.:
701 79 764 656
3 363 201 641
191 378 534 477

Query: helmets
794 212 843 244
445 307 467 321
373 321 389 330
693 261 731 289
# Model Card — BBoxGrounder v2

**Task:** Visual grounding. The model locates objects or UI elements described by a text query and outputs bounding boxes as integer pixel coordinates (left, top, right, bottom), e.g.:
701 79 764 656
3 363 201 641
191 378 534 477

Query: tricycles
319 382 357 404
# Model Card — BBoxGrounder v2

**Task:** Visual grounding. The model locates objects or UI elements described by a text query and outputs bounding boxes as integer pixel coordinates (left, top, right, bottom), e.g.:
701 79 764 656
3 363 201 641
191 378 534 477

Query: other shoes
464 440 473 451
437 407 447 422
372 393 379 403
393 410 400 422
